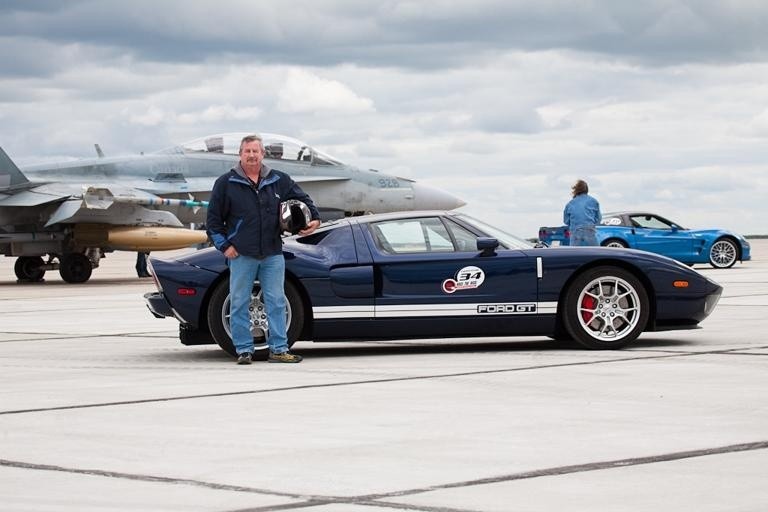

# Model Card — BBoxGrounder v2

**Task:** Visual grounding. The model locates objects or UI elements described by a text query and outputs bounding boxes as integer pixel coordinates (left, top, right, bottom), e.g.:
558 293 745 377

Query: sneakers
267 352 302 363
237 352 252 364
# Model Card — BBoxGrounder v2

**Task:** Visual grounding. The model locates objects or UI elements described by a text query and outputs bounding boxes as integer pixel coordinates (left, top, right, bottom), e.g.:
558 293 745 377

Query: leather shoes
145 272 151 277
139 274 148 277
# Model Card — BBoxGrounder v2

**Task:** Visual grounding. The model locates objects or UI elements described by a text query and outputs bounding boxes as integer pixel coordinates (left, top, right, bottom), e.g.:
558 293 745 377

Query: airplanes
1 130 467 284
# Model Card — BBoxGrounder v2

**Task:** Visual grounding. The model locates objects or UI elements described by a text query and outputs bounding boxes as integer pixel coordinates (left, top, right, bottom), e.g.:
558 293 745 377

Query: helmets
278 199 312 237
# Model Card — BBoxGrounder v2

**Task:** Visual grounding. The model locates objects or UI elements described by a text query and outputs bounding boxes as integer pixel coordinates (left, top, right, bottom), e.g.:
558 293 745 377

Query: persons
562 179 602 247
204 135 323 366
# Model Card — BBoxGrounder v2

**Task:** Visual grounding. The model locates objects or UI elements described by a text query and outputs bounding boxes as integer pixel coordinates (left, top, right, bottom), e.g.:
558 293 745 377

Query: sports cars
536 210 751 269
142 209 724 362
